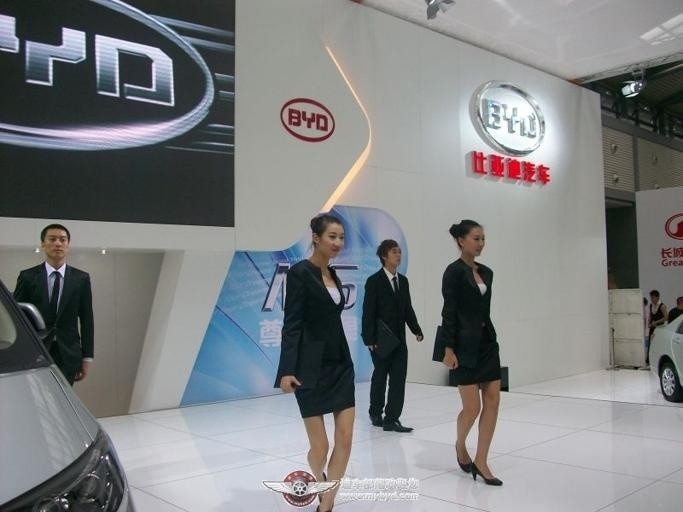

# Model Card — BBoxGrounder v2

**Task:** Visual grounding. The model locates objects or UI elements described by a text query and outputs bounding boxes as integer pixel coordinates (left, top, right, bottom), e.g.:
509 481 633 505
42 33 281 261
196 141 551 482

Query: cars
1 280 138 512
649 314 682 404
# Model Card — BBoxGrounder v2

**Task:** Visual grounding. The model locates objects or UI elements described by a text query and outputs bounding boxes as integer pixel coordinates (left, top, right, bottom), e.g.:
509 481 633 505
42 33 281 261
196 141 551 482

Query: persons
634 290 683 369
441 218 504 485
360 240 425 432
274 214 355 512
12 224 94 388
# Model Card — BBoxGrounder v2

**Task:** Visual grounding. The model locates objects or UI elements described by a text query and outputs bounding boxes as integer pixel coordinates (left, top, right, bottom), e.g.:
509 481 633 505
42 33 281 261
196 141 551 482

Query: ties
51 273 60 311
393 277 398 292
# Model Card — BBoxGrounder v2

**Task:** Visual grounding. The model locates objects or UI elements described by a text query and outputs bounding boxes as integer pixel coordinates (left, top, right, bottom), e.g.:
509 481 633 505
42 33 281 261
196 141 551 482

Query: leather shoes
383 419 413 432
369 412 383 426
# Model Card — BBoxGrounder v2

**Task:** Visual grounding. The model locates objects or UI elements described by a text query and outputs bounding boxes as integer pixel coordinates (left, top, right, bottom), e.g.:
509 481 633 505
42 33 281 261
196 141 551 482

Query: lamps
425 0 456 19
622 68 647 99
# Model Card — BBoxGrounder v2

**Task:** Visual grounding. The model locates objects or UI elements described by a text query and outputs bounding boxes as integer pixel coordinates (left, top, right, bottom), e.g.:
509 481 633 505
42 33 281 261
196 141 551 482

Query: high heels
455 442 472 472
472 462 503 486
